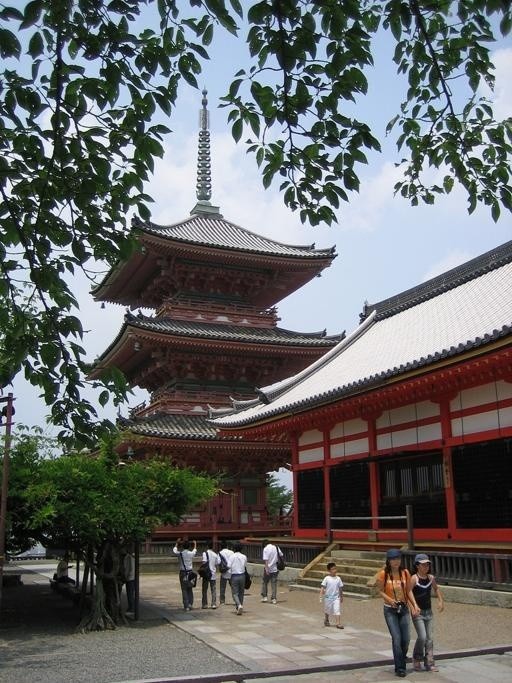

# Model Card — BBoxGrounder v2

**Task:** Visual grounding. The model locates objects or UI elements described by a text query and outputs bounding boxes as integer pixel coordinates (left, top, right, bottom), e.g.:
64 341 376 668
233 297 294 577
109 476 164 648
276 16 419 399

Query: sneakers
185 604 225 613
261 597 276 604
337 624 345 629
426 665 439 671
414 659 420 669
324 620 330 626
237 605 243 614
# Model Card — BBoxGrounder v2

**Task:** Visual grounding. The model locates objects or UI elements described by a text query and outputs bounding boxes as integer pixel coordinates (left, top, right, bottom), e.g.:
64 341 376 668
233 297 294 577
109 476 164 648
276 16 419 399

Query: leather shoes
398 671 406 676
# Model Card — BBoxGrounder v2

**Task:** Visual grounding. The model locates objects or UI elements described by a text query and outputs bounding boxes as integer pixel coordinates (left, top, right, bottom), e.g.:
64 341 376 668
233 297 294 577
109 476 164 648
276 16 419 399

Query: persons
226 542 248 615
374 548 420 676
406 553 444 672
173 536 197 611
217 541 235 606
121 547 136 615
201 543 220 609
319 562 344 629
260 538 285 604
54 553 76 584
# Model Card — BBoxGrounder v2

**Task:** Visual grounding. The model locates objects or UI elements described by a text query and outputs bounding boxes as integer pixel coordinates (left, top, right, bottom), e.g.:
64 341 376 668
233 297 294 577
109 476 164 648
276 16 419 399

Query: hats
386 549 402 557
413 553 432 564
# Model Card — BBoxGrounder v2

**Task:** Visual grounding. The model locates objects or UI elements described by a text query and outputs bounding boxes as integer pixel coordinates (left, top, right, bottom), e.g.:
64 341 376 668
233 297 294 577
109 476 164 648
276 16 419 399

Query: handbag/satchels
245 570 251 588
219 556 229 572
197 561 212 578
277 556 284 569
182 572 197 586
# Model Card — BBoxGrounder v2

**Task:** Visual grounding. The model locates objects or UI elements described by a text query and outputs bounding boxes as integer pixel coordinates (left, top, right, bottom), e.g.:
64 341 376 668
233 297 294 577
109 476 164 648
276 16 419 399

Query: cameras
396 601 407 615
